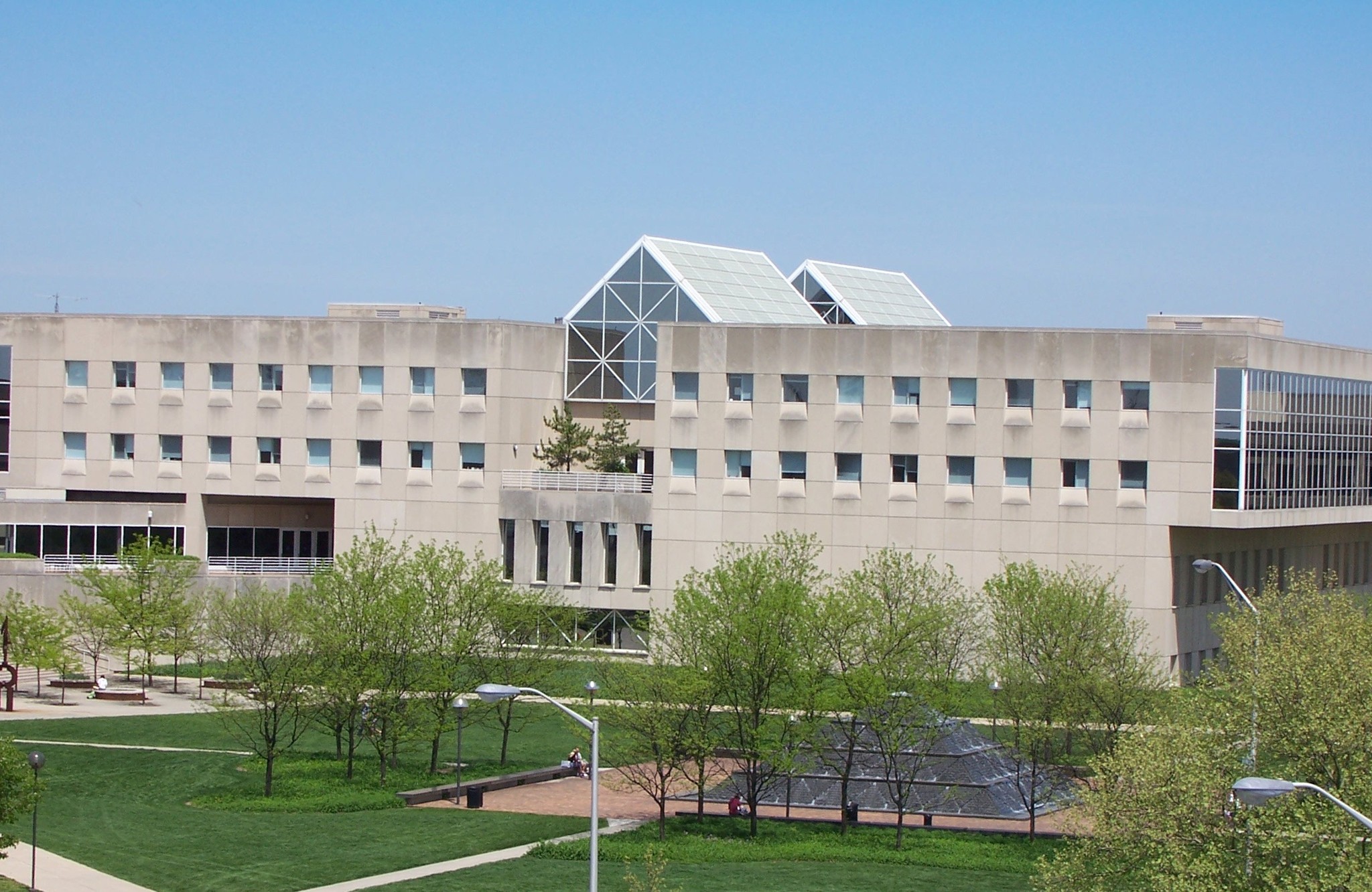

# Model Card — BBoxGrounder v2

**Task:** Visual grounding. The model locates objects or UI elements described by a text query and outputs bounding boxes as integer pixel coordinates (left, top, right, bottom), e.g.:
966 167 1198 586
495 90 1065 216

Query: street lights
988 681 1003 742
452 696 469 805
1192 559 1261 881
584 681 600 706
27 751 46 888
475 683 598 892
1233 776 1372 833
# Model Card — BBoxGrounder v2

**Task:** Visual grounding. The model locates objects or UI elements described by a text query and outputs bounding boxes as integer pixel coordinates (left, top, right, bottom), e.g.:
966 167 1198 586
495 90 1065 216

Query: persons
729 795 741 815
568 748 587 777
92 675 108 691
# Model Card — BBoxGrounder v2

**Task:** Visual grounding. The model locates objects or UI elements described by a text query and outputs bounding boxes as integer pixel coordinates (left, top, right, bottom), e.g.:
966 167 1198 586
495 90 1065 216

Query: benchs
396 762 577 805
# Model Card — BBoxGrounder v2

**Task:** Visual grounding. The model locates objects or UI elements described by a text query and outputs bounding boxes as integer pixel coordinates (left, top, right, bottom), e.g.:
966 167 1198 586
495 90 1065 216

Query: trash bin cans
924 814 932 825
467 786 483 808
841 804 857 822
